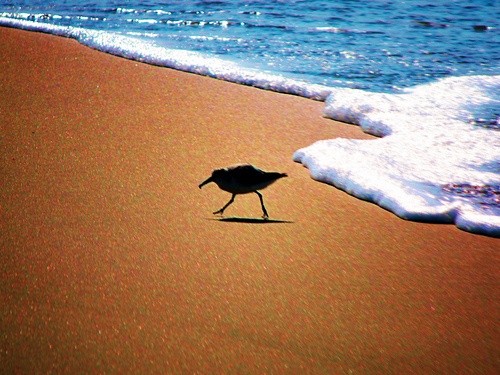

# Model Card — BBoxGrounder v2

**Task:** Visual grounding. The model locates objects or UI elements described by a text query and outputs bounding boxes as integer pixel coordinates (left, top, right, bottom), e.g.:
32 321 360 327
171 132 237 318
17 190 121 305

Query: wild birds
198 164 288 220
